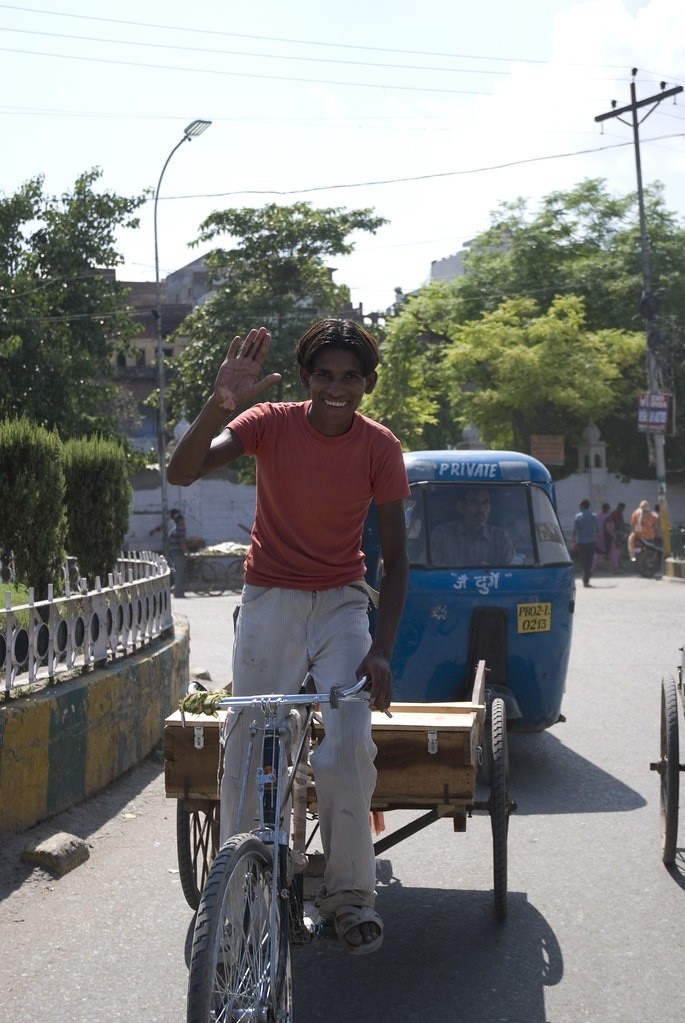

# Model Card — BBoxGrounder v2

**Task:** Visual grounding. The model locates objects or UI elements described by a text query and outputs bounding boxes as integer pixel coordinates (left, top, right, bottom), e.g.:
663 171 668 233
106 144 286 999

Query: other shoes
584 584 590 587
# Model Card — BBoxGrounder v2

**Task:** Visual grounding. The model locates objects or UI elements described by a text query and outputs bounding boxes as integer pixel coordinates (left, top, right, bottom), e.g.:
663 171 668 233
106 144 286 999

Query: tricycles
165 551 247 596
163 658 517 1023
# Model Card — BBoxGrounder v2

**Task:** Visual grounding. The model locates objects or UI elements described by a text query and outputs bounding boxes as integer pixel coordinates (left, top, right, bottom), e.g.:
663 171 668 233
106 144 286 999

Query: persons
148 510 178 588
418 485 517 568
611 502 625 531
165 318 410 973
628 499 659 562
167 514 190 597
625 507 653 538
590 502 623 575
572 499 603 587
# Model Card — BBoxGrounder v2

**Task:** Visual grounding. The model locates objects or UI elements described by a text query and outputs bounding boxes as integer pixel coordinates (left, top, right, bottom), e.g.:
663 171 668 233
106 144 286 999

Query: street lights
153 120 214 556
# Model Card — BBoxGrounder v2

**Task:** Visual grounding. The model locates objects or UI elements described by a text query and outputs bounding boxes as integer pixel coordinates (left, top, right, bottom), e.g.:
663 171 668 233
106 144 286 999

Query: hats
640 500 650 510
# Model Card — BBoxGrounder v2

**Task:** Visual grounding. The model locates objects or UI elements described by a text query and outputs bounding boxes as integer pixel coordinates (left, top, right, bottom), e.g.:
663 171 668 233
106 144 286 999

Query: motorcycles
636 523 685 578
357 449 575 736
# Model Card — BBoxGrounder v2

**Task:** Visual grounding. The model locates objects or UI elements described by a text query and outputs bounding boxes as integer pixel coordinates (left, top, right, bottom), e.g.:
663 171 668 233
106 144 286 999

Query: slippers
332 905 383 955
216 925 238 967
